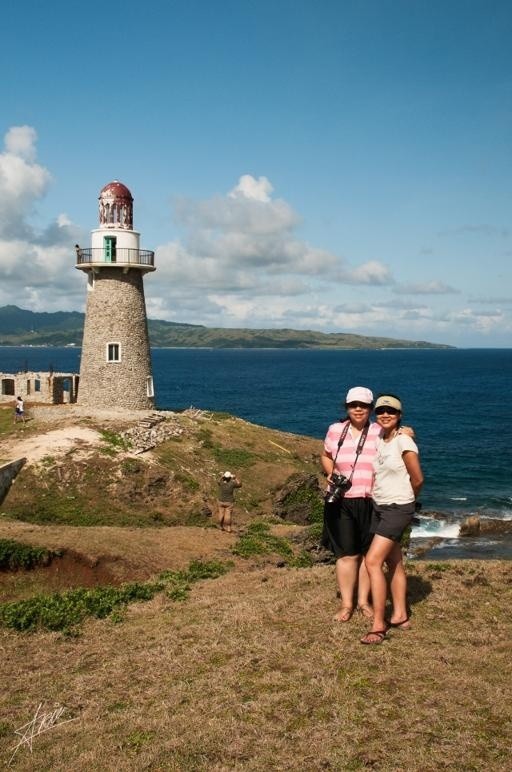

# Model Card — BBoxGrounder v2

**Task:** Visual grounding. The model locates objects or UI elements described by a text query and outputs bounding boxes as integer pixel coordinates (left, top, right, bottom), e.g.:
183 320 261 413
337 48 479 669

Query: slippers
332 607 354 623
357 606 374 619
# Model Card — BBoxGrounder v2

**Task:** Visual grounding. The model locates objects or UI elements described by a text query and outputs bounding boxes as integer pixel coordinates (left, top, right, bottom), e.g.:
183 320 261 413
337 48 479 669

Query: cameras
324 472 353 508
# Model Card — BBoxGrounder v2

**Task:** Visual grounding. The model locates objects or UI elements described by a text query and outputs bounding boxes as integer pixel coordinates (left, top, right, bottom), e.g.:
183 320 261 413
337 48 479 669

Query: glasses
374 408 398 415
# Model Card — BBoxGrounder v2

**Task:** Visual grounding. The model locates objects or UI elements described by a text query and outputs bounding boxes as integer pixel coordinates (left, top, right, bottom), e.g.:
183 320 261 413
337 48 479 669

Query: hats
345 387 374 405
373 396 402 413
224 472 235 478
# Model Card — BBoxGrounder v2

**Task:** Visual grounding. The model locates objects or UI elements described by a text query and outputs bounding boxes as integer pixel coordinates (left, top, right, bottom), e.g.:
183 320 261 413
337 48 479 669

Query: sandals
361 630 387 645
384 616 411 630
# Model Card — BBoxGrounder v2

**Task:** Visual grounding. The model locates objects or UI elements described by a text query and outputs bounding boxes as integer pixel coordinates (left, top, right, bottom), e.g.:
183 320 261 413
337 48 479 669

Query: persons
13 396 26 426
74 243 84 264
320 385 416 624
360 393 424 644
214 470 242 533
